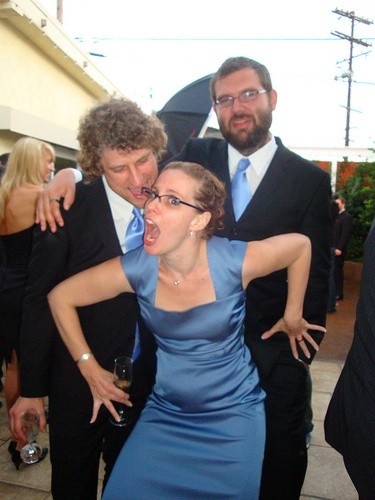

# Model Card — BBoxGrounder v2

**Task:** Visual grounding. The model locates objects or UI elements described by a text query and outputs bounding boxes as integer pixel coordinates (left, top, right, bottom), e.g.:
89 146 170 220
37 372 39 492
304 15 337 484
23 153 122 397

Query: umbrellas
151 71 220 174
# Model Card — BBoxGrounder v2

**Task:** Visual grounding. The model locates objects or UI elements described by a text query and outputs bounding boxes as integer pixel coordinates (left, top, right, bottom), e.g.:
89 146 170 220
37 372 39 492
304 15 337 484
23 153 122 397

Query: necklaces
160 252 203 286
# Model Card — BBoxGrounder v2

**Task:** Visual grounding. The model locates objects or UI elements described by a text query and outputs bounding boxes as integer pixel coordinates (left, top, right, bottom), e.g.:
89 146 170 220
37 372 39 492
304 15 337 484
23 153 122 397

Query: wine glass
18 409 42 464
108 356 136 429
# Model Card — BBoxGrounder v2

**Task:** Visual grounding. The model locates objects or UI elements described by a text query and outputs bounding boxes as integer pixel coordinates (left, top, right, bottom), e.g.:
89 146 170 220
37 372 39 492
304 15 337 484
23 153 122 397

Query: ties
230 158 253 222
125 206 145 364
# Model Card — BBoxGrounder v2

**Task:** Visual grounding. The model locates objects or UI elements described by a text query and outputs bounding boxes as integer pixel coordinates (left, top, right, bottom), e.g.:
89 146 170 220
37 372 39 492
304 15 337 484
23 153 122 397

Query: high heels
8 438 48 471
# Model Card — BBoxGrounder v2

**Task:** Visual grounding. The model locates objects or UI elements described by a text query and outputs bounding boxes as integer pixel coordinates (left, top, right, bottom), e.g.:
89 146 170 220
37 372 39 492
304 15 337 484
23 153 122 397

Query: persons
0 152 10 168
323 219 375 500
36 56 334 500
45 161 326 500
333 194 351 301
0 137 55 474
326 195 340 314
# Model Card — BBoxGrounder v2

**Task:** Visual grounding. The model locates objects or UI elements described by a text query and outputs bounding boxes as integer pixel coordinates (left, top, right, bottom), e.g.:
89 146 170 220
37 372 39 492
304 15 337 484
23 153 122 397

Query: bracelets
74 350 92 364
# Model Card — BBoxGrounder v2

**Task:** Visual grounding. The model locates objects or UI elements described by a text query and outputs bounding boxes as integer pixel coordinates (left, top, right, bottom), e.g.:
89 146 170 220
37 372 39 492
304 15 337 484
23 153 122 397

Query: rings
49 199 61 205
298 339 303 342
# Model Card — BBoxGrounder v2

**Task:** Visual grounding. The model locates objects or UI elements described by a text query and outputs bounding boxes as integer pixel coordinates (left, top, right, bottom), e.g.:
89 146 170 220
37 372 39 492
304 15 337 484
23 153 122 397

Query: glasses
141 186 205 214
214 89 268 108
